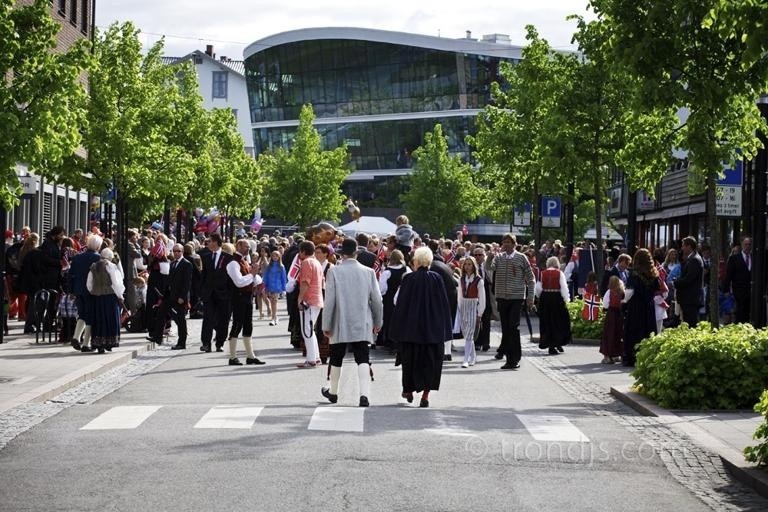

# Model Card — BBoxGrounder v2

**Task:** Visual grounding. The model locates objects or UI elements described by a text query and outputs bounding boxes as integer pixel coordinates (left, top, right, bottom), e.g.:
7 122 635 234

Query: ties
210 253 216 269
172 260 178 274
744 253 750 268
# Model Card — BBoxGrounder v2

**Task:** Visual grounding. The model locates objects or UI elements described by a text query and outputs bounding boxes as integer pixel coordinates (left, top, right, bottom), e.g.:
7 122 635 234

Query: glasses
474 254 485 257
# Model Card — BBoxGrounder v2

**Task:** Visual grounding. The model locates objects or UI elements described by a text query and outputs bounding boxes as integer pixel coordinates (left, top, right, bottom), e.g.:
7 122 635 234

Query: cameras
300 300 309 309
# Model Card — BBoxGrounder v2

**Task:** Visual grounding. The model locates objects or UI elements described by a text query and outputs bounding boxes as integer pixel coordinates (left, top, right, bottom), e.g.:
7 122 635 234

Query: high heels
402 390 413 403
420 400 429 407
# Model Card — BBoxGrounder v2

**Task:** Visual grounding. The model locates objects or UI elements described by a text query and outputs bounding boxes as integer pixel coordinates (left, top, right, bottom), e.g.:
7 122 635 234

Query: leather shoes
229 358 243 366
171 344 186 350
147 337 161 345
322 386 338 403
461 361 469 368
468 360 476 366
358 395 369 407
512 362 520 368
246 358 266 364
501 363 513 369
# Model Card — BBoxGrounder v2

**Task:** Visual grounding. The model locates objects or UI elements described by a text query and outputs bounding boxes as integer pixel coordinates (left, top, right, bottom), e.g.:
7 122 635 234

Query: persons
0 214 767 407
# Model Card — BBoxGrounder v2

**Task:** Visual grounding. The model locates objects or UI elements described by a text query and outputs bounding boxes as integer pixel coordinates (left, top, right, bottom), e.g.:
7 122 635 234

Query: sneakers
81 346 91 352
548 347 558 354
601 356 614 364
70 339 81 350
556 346 564 353
